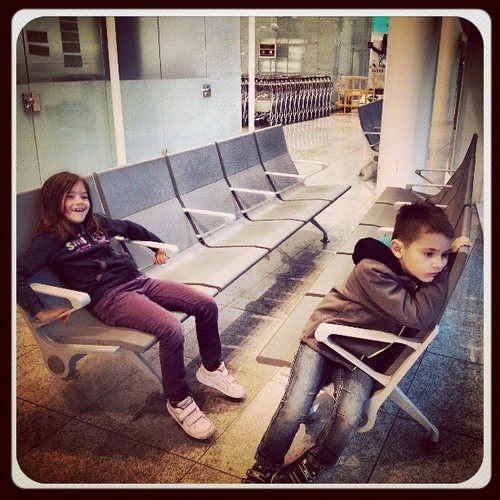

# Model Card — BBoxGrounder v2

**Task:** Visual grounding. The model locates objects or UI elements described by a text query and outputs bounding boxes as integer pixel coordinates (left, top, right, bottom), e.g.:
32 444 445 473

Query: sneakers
195 361 245 398
241 461 284 483
166 396 215 439
271 448 321 484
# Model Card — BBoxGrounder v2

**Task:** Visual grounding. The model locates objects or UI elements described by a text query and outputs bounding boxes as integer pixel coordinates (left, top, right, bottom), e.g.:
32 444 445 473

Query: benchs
257 133 478 441
358 99 384 176
15 124 352 442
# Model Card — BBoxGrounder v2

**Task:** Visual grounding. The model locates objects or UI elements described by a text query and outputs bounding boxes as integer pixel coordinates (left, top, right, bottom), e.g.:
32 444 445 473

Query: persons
244 198 472 484
17 172 246 438
